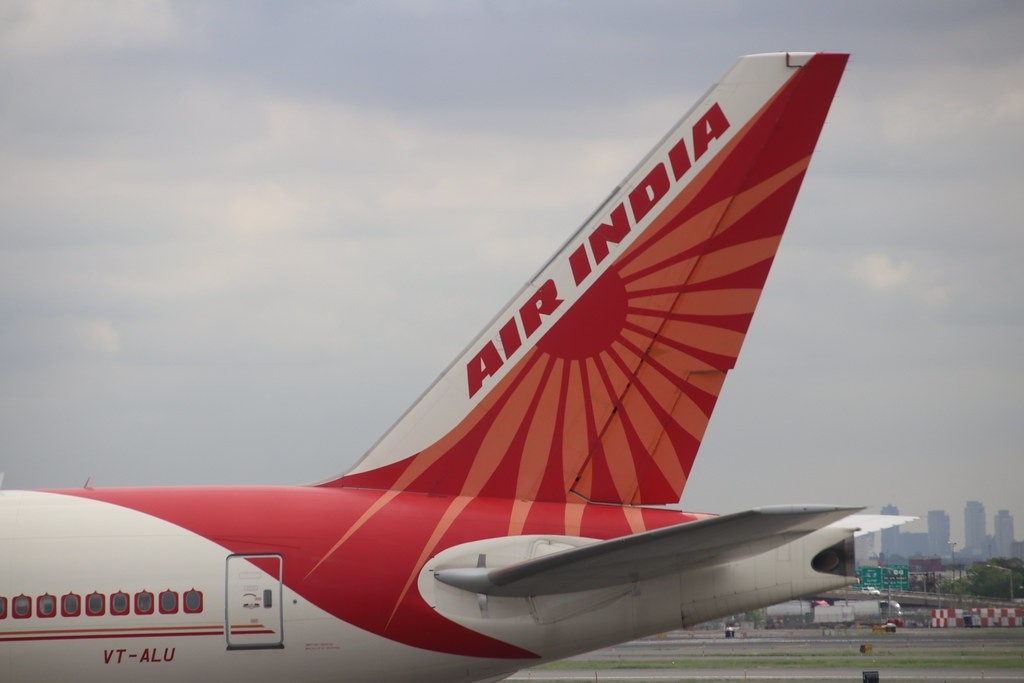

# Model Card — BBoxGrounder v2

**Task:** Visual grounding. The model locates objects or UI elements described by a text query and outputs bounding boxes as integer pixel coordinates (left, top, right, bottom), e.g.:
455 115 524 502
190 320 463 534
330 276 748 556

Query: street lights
986 563 1015 603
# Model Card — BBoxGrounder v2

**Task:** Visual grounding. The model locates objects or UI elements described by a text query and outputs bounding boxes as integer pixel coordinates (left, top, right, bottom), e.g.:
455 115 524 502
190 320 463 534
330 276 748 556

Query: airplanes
1 51 925 683
